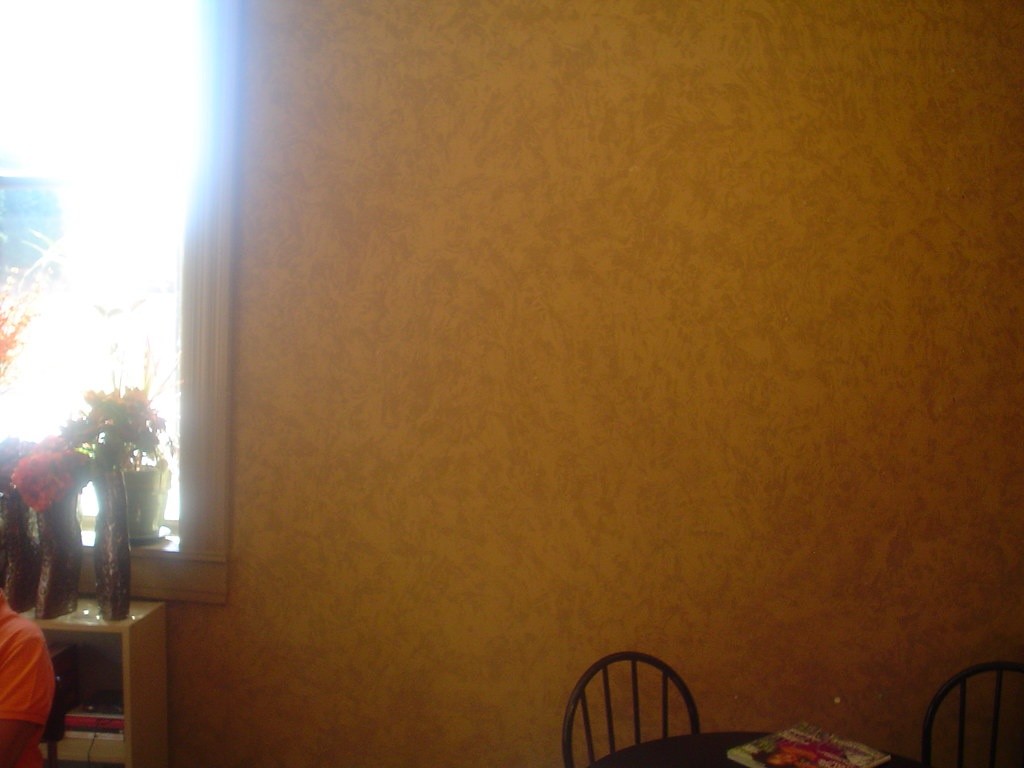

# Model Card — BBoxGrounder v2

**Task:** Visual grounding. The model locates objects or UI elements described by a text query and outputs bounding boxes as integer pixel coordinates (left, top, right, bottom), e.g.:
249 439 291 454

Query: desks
585 732 923 768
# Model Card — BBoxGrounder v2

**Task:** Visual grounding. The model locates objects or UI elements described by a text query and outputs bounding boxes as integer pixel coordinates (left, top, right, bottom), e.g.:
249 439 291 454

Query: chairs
922 661 1024 768
562 650 701 768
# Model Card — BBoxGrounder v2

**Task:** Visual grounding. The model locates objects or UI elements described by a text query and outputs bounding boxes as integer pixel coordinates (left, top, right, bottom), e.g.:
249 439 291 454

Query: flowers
0 386 166 512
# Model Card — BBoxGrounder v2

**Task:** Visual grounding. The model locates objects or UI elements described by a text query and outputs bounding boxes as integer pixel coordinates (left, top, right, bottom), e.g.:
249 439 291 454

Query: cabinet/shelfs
16 600 168 768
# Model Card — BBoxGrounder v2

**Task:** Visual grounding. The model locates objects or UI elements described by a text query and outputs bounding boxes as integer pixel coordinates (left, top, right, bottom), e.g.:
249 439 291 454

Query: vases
2 497 41 613
35 502 83 619
92 470 132 622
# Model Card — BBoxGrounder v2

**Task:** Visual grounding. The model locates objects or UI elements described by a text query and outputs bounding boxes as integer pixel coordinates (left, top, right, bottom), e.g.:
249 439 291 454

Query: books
727 720 891 768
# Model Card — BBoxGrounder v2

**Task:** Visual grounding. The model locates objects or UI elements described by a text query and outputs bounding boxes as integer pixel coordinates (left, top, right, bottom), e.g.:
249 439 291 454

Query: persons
0 544 56 768
751 738 860 768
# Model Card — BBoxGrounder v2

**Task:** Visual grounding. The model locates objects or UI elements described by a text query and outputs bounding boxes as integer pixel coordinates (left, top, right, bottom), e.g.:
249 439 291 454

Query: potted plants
79 337 177 537
